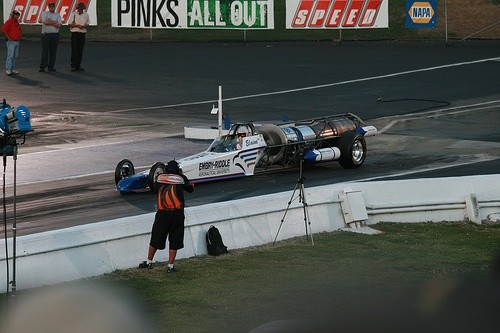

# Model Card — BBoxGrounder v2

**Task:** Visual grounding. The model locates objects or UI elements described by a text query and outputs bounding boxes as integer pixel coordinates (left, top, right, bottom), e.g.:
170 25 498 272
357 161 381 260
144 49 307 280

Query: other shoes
39 67 45 72
71 65 76 72
6 70 12 75
13 70 20 74
77 66 84 71
48 66 56 72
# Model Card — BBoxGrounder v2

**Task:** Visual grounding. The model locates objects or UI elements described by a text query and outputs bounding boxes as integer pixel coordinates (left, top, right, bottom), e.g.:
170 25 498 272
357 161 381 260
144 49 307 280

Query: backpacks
206 225 231 256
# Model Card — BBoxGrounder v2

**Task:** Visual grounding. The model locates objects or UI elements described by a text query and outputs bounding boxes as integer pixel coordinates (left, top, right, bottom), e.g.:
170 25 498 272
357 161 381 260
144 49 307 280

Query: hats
12 10 21 15
48 2 55 5
76 3 86 9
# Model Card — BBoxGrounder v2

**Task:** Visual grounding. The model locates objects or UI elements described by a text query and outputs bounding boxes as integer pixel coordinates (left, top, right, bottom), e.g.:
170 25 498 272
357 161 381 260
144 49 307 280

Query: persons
2 10 22 74
39 2 62 73
69 3 89 71
139 161 195 271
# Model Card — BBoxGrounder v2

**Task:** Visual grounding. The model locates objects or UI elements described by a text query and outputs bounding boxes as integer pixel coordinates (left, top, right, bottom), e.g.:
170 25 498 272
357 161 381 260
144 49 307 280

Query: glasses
80 9 83 10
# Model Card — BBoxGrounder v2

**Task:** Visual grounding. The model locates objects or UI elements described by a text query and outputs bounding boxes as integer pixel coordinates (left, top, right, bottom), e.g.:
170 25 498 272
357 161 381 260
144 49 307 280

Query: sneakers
167 265 176 273
139 260 153 269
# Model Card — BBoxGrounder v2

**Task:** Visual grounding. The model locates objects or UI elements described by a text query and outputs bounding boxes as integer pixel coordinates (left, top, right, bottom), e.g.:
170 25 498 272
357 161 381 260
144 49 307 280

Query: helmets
165 160 182 174
